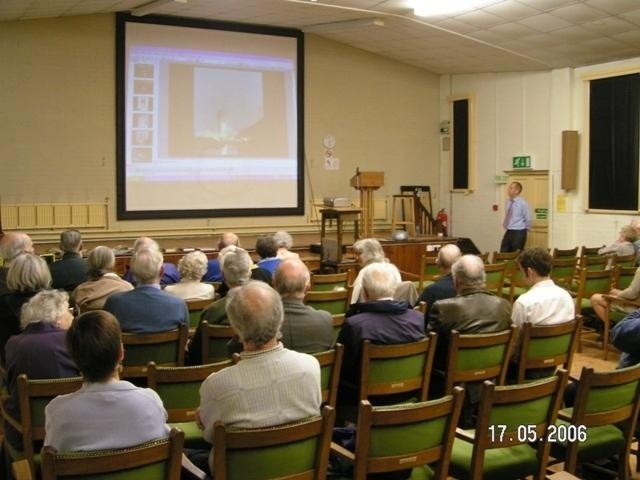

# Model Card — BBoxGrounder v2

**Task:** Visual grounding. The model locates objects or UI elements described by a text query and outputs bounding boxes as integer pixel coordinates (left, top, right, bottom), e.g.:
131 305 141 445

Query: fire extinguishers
438 208 448 236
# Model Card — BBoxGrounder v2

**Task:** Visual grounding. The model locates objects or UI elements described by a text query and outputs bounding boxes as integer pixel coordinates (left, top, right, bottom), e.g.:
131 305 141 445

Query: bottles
416 223 421 238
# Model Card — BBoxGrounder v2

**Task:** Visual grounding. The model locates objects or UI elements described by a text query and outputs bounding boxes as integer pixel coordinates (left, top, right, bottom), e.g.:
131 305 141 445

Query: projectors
324 197 351 207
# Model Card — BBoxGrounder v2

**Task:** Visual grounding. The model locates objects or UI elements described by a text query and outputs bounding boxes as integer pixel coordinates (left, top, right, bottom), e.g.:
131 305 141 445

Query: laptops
323 239 356 263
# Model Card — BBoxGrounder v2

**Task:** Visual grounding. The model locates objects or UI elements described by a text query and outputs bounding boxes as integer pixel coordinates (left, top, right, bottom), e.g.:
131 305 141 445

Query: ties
503 200 515 228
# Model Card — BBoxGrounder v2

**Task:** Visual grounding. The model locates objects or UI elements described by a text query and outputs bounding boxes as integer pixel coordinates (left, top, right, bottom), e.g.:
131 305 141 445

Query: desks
319 208 362 245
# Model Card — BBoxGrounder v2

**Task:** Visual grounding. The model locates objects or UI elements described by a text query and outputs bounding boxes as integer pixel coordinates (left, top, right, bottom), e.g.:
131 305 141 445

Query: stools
391 194 416 238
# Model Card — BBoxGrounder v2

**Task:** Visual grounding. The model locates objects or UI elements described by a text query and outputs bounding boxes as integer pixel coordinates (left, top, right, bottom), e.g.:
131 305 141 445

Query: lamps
131 0 187 17
302 18 384 32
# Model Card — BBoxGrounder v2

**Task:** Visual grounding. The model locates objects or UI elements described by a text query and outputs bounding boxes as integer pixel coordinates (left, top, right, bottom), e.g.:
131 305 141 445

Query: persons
508 247 575 362
590 222 639 368
1 221 332 379
39 314 171 462
187 278 323 439
415 243 513 422
325 237 426 473
498 180 532 253
